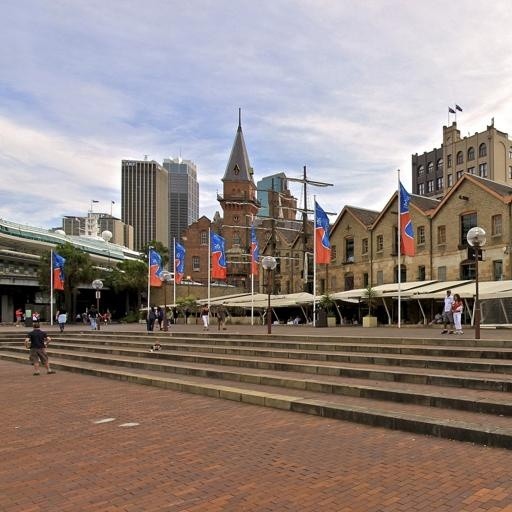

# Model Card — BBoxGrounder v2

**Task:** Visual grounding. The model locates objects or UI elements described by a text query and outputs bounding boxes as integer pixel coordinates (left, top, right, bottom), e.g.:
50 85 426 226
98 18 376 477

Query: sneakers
441 329 464 335
33 371 55 375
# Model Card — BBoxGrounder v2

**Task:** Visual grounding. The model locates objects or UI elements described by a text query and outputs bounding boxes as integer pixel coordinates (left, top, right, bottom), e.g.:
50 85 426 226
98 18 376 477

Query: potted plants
319 289 337 328
362 285 378 328
185 294 197 323
175 295 185 324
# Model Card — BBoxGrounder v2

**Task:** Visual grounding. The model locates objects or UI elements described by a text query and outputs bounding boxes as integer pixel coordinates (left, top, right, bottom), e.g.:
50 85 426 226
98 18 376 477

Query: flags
92 200 99 203
449 107 456 113
112 201 115 204
455 104 462 112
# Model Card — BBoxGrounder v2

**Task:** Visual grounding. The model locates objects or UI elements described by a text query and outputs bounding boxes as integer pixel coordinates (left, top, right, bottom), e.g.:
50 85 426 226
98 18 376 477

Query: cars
181 278 236 287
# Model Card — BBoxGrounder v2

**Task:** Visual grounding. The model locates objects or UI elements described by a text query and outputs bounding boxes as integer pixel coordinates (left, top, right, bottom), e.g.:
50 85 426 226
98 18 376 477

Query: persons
337 312 466 326
16 304 111 376
451 294 464 335
146 302 232 331
439 289 454 335
287 310 319 325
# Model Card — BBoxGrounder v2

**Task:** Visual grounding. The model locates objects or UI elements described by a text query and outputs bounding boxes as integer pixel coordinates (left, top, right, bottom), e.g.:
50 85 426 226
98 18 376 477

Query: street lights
466 227 486 340
92 280 103 330
111 200 115 216
159 271 171 332
262 256 277 334
